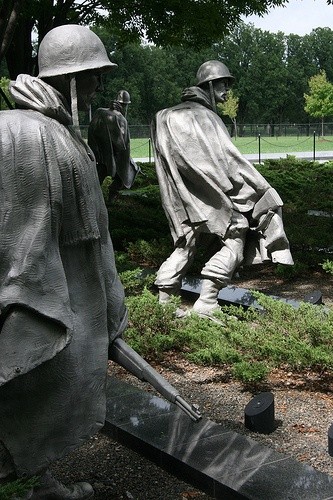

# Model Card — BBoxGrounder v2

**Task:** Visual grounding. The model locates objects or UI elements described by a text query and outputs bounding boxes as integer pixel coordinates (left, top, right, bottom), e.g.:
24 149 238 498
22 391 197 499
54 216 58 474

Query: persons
0 24 133 500
86 89 141 208
153 60 295 330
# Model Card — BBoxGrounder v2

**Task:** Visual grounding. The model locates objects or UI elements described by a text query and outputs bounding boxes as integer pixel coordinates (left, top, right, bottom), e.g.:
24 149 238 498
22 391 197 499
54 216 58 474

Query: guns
108 337 202 422
139 170 148 178
235 211 275 266
